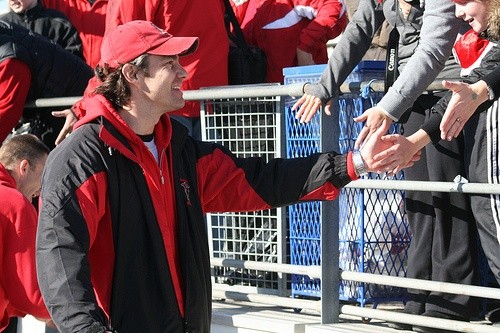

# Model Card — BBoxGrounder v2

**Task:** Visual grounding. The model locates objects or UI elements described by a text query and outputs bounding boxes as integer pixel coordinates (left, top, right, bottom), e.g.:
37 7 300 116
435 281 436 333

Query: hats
101 20 200 74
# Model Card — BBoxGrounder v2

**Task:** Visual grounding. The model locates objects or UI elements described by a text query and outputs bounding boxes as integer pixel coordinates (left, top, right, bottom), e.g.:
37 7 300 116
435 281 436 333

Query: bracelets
353 150 367 176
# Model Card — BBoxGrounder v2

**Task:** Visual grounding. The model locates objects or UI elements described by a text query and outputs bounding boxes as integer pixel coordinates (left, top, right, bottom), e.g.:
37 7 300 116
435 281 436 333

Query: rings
457 119 461 123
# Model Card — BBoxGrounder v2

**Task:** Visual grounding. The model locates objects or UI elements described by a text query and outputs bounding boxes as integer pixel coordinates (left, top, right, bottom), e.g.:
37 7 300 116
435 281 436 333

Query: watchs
484 82 495 101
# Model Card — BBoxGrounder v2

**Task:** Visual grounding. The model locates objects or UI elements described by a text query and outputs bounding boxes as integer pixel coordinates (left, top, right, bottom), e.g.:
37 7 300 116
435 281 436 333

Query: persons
0 0 349 152
0 134 52 333
292 0 500 333
33 19 421 333
